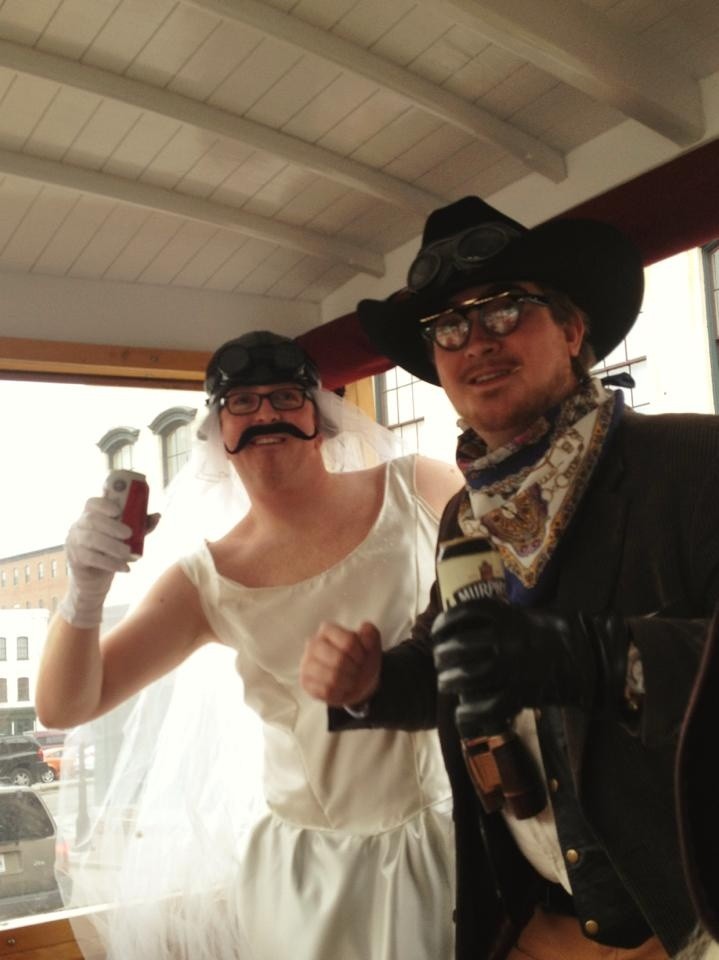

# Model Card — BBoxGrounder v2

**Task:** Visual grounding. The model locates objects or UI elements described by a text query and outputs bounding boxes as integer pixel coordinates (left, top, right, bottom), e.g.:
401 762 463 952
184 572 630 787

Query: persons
301 197 719 960
33 331 470 960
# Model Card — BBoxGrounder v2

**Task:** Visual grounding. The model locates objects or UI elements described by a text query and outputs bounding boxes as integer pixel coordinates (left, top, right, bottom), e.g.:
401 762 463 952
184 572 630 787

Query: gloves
59 497 158 628
431 568 600 734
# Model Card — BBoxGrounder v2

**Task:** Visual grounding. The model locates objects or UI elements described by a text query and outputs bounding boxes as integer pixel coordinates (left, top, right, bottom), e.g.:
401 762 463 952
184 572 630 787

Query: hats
355 195 645 387
203 332 318 405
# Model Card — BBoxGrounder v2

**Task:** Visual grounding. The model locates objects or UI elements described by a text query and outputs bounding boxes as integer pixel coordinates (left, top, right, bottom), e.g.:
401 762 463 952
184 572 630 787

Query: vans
0 789 73 924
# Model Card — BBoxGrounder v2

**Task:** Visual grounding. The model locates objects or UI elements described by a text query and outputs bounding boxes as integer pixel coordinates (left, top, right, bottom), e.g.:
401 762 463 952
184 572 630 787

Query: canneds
436 536 509 612
102 470 149 561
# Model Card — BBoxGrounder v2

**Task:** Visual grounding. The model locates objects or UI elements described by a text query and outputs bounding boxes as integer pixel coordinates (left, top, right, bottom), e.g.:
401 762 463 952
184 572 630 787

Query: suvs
0 731 66 787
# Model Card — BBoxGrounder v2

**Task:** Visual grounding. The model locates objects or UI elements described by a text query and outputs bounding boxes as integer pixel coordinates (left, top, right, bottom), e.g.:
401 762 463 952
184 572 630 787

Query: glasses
410 292 550 351
219 388 311 415
